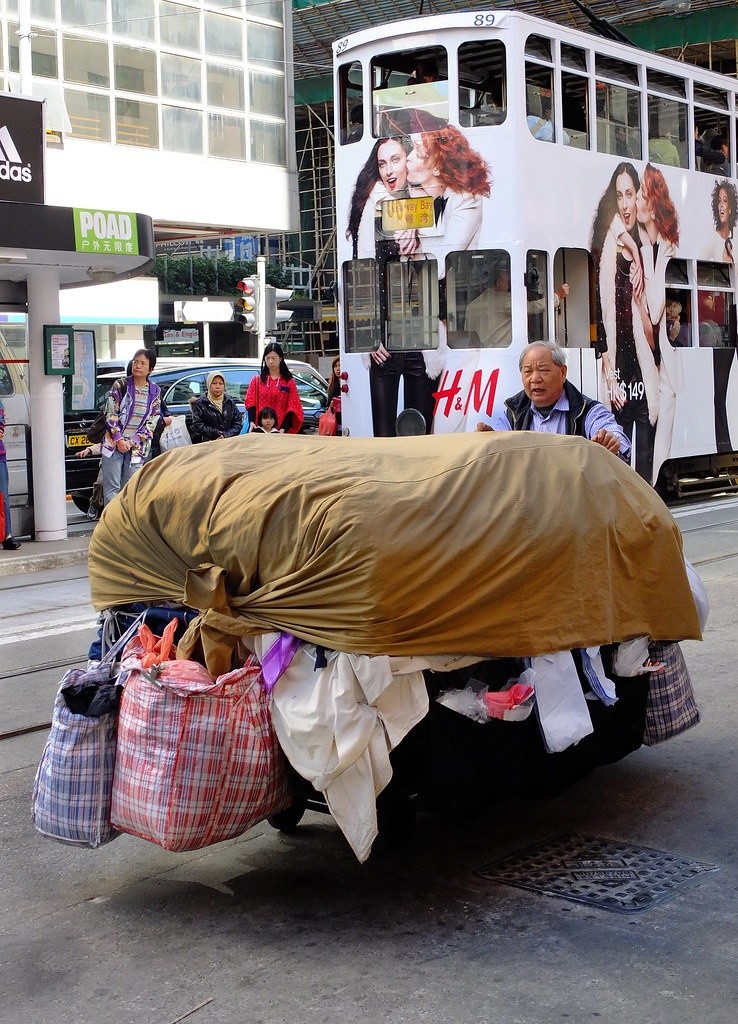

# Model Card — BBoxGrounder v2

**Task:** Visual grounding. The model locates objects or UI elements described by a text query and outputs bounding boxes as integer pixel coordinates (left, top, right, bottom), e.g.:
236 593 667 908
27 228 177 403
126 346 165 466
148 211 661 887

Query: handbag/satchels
525 637 701 776
108 629 292 853
319 407 337 435
31 612 148 850
160 414 192 453
86 378 127 444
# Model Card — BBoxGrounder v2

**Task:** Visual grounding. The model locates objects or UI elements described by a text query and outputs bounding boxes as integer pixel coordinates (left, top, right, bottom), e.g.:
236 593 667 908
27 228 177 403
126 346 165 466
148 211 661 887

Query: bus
332 11 738 499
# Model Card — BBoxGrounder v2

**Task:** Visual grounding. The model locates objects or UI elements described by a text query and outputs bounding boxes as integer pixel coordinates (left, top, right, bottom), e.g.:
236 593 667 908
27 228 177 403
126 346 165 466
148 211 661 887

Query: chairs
677 319 724 348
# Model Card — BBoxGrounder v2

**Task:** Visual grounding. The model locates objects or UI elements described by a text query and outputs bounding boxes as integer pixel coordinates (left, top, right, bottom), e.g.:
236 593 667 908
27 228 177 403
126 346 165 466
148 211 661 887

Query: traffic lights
265 285 294 333
237 278 260 333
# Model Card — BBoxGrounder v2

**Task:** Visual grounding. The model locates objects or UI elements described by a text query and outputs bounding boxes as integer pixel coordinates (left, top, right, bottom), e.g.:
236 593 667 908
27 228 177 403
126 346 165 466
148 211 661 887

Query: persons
706 180 738 453
382 105 411 137
326 355 342 436
527 107 570 145
463 267 570 348
681 123 732 177
666 297 726 347
347 124 492 437
244 343 304 434
186 371 243 445
590 162 680 486
649 125 680 167
0 399 22 551
75 349 173 521
406 65 443 133
475 340 631 463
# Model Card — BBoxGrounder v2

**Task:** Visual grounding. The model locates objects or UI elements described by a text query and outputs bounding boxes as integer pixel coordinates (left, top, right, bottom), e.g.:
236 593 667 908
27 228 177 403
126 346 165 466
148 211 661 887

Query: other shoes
2 537 21 550
86 502 99 520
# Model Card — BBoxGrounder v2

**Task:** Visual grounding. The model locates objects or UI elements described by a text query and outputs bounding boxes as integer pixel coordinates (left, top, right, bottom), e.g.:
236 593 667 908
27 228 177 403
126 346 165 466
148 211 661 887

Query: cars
64 355 331 518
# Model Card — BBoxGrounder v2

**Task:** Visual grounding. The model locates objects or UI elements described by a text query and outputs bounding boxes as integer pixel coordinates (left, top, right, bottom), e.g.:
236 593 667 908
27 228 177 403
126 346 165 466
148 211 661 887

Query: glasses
131 360 147 366
